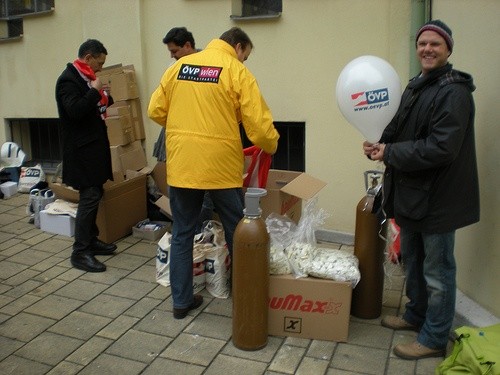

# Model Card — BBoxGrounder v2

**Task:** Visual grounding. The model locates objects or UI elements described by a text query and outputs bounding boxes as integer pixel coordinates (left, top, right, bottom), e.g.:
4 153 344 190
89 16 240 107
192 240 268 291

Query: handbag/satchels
26 187 56 229
155 219 233 300
434 322 499 375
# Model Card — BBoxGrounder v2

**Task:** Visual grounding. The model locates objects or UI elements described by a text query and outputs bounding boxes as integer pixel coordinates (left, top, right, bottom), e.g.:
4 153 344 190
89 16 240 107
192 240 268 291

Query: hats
415 19 454 57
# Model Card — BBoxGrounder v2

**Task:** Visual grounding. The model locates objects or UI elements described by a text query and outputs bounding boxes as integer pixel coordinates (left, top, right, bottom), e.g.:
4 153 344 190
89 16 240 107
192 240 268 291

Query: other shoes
380 315 423 330
173 294 203 319
393 341 447 360
92 238 118 254
70 253 107 272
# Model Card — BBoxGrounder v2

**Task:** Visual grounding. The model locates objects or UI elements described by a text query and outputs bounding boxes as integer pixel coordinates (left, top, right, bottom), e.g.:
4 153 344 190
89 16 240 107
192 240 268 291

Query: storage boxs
243 169 328 236
39 63 172 241
268 274 352 343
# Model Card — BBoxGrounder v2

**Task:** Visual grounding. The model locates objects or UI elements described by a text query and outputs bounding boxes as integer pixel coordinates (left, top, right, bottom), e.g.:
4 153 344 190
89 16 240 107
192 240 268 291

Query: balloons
335 54 402 146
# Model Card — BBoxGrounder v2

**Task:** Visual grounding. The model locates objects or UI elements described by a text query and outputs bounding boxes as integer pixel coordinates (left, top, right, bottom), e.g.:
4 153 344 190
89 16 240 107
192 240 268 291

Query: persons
148 28 279 319
163 26 214 233
361 18 481 358
56 39 118 273
